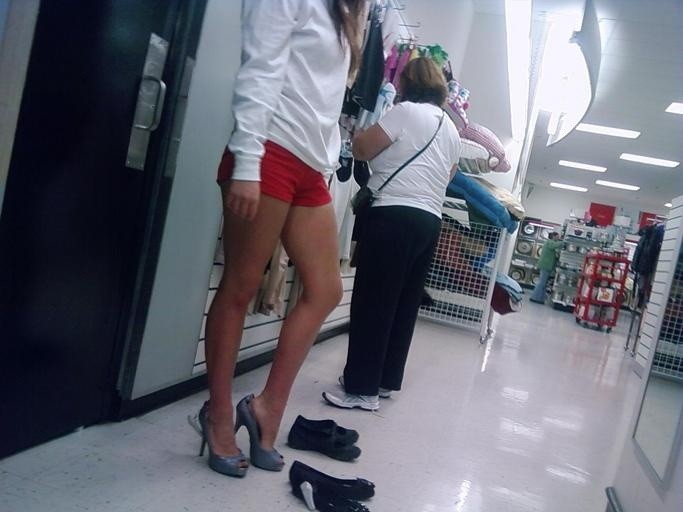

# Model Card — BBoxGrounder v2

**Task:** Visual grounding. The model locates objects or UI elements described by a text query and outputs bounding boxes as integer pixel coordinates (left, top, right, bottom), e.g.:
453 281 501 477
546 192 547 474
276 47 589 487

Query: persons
319 57 463 413
528 231 564 304
196 0 368 478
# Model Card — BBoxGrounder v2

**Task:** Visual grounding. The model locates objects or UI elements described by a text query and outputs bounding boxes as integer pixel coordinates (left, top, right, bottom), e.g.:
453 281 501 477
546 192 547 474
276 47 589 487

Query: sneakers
339 375 392 399
322 391 380 411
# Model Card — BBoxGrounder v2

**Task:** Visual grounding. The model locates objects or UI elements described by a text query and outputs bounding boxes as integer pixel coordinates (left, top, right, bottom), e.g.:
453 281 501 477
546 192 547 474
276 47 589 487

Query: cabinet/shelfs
550 218 627 310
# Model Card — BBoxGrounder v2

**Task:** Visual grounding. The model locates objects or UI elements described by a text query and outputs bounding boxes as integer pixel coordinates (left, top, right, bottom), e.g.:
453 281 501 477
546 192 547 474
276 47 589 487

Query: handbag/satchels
350 185 374 214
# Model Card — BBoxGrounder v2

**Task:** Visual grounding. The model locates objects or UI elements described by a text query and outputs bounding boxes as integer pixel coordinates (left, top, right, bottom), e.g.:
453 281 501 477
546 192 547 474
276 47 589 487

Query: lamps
544 0 603 145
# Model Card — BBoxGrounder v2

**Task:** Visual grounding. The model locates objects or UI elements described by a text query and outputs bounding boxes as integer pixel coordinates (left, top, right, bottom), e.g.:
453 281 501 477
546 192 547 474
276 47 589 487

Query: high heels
234 393 284 471
199 398 248 478
290 460 374 512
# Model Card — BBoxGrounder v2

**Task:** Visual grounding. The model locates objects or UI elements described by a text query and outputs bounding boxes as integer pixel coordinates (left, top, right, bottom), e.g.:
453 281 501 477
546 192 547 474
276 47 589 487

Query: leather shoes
286 416 361 460
